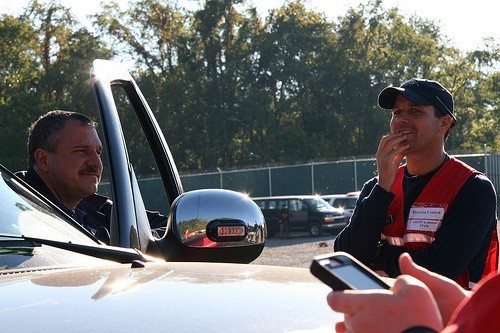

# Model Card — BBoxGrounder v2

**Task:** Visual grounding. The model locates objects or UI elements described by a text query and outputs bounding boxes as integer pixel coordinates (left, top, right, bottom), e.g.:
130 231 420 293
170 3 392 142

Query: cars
319 194 359 219
0 60 343 333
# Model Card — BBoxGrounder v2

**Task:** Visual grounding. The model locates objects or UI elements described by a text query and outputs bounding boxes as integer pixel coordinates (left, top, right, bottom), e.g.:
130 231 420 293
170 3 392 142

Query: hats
377 78 457 122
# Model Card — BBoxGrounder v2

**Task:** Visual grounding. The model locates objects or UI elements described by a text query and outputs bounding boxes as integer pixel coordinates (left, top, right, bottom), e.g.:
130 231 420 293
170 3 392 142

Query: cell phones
310 250 393 292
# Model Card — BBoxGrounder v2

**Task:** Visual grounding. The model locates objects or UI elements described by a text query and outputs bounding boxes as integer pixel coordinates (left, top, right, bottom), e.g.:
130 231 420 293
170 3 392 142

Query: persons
246 221 266 243
328 253 500 333
333 78 500 292
279 204 290 239
14 110 168 246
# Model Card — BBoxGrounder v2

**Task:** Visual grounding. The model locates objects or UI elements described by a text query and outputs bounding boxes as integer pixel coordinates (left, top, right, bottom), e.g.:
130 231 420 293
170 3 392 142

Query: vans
250 195 348 238
